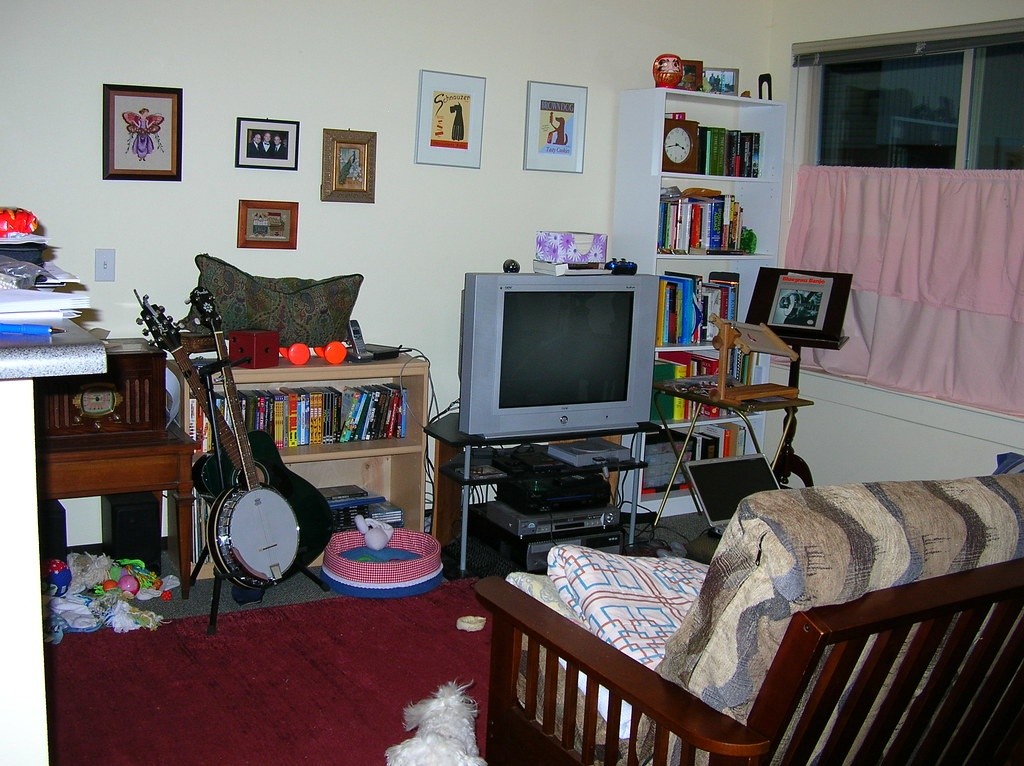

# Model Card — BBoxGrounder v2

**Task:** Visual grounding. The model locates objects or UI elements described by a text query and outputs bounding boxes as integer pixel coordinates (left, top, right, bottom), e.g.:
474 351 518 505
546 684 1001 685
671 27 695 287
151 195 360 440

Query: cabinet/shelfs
165 352 429 581
609 87 789 518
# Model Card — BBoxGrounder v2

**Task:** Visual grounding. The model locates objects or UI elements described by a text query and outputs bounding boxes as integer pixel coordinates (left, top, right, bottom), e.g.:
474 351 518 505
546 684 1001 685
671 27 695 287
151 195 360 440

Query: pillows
172 253 364 348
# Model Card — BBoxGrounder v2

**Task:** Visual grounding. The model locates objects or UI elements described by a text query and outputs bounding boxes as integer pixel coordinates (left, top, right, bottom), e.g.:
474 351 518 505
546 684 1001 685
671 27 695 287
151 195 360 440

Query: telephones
346 320 374 363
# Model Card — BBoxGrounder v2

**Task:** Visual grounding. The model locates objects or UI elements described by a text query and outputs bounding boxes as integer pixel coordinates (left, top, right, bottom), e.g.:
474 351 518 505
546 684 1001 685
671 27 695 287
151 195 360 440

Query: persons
247 133 288 159
709 73 720 92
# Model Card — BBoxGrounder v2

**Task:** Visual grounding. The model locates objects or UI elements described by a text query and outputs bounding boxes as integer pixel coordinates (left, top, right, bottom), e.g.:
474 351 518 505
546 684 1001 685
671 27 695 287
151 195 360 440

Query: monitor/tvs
459 273 660 439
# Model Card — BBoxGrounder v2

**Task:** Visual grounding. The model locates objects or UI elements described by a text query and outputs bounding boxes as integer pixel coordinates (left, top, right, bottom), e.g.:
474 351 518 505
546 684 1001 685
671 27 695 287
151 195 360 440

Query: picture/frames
234 117 300 171
413 68 487 170
102 83 183 182
703 67 740 96
320 128 377 203
656 60 703 91
237 199 300 250
522 80 588 174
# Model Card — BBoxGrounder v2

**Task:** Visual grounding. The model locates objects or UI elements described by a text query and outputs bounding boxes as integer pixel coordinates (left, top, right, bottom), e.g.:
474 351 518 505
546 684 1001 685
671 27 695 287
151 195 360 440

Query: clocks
662 118 700 174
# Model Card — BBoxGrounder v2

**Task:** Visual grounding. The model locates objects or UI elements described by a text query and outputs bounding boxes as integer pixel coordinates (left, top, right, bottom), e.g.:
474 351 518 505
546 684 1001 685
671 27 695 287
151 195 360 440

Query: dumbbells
278 340 347 366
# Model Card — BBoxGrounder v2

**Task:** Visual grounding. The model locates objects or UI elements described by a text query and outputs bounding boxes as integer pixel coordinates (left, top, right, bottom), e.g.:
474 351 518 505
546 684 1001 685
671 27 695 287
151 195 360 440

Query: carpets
45 576 493 766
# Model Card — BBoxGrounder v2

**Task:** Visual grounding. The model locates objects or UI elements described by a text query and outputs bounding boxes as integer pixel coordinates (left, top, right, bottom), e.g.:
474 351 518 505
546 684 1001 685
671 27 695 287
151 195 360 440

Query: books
655 270 740 347
686 422 747 461
649 347 758 423
657 194 744 254
188 383 407 454
697 126 760 178
318 484 405 532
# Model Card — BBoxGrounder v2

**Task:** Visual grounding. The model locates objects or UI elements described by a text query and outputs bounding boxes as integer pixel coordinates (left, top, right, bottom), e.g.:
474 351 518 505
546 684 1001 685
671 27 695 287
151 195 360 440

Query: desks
652 380 814 528
37 418 197 602
424 413 663 583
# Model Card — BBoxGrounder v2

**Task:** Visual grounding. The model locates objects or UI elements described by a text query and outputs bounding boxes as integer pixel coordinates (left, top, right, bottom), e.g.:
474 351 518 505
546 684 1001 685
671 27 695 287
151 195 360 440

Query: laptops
683 452 782 535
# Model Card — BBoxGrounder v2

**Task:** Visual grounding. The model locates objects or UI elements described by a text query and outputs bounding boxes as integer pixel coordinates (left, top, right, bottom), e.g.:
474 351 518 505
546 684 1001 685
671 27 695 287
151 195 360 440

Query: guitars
133 288 335 586
186 286 300 591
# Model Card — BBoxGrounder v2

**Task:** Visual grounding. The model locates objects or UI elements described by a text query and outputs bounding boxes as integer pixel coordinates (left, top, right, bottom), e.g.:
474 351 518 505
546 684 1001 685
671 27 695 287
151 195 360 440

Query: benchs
466 453 1024 766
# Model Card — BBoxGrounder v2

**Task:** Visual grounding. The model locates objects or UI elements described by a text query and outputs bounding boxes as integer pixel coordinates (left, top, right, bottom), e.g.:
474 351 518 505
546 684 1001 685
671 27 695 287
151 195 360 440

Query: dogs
385 680 488 766
